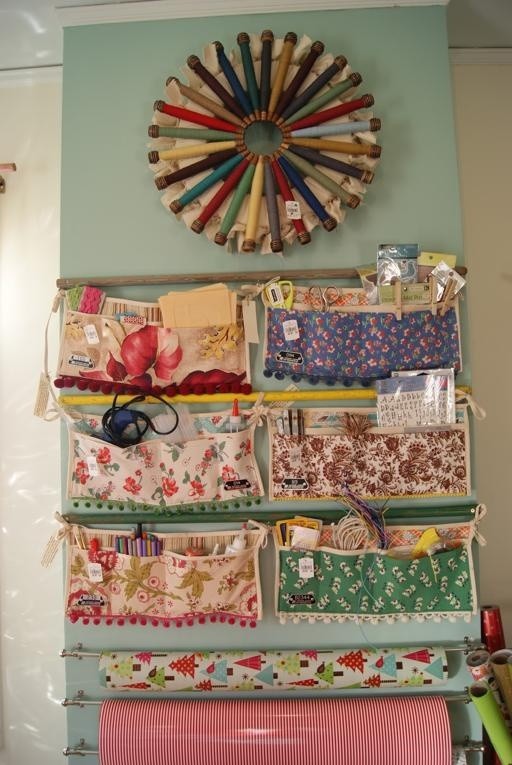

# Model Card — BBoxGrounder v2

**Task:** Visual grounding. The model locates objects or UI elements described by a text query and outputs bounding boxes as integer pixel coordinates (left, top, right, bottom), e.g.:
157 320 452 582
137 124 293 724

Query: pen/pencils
76 527 98 550
114 522 160 557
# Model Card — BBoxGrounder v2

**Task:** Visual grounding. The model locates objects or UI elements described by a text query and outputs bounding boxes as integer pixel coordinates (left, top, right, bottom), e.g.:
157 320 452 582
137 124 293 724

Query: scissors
309 284 340 313
265 280 293 311
